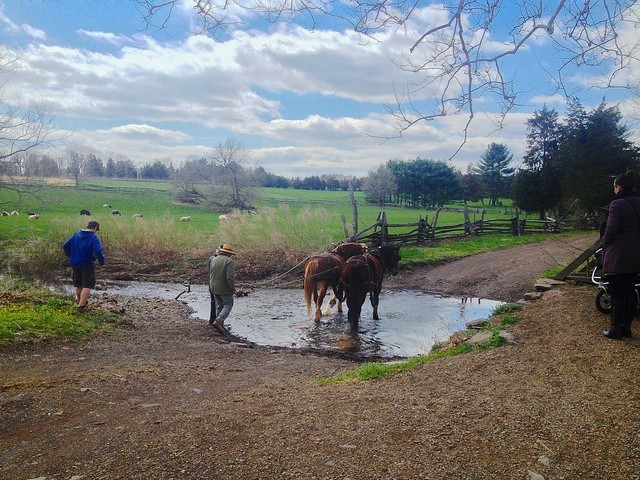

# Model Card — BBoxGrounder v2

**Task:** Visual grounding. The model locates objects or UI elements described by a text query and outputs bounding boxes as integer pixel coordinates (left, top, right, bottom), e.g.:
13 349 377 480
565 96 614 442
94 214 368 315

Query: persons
207 245 223 327
600 170 639 341
62 220 105 310
209 243 236 335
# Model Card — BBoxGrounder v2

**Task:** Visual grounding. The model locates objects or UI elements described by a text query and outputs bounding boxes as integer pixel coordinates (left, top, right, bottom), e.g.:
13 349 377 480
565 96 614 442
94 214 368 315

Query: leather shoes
603 330 623 339
624 329 631 339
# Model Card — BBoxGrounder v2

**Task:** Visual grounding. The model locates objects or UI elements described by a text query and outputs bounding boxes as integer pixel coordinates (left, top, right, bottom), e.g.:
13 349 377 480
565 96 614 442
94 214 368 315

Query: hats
215 245 223 250
88 221 100 231
219 244 236 255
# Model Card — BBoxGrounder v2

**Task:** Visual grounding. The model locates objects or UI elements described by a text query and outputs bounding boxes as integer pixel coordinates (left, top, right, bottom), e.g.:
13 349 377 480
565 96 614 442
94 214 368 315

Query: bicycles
592 238 640 313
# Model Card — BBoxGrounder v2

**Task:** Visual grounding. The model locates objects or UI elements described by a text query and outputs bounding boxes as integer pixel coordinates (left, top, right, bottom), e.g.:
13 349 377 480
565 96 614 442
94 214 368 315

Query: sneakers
75 298 89 305
212 319 225 336
77 304 94 314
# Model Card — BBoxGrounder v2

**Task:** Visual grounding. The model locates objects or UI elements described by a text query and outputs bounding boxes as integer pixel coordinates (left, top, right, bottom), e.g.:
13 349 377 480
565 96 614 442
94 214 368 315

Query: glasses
611 185 618 189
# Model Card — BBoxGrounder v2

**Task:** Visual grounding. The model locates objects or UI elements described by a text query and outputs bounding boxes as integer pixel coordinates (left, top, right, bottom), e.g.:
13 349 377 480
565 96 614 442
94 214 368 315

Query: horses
341 240 403 329
303 241 368 323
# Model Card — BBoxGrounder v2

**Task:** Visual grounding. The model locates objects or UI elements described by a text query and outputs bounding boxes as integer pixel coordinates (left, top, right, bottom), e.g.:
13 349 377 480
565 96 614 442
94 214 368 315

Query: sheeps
29 214 39 220
111 210 122 217
3 211 10 217
219 215 228 222
180 216 192 222
11 211 19 216
80 209 90 216
